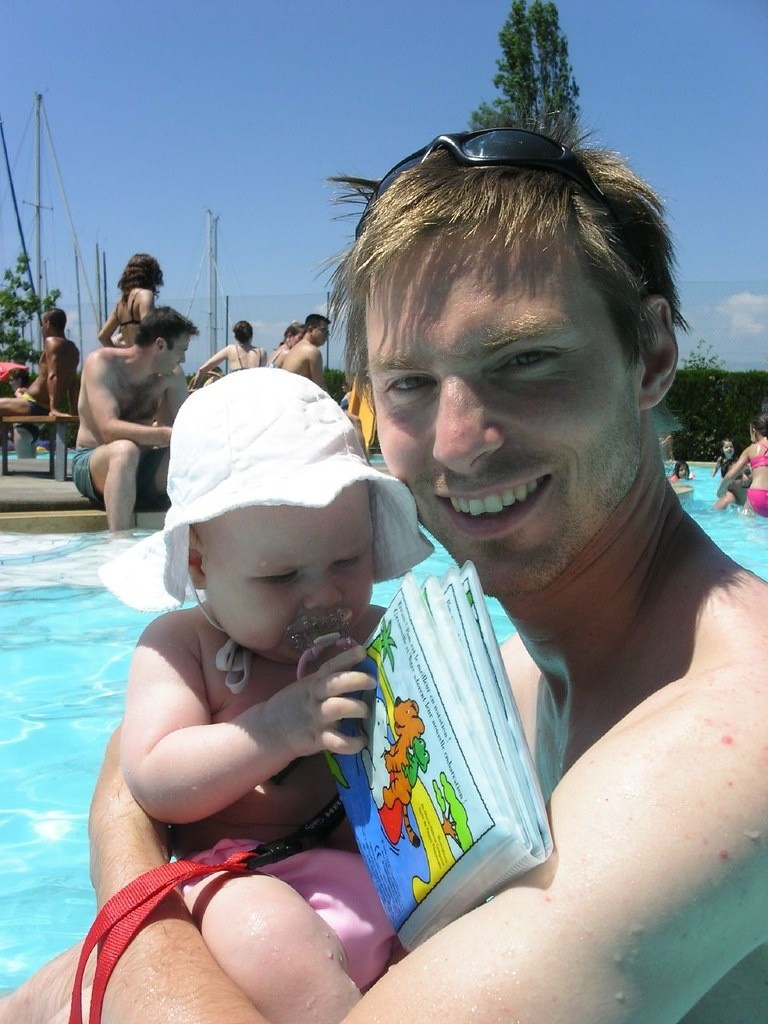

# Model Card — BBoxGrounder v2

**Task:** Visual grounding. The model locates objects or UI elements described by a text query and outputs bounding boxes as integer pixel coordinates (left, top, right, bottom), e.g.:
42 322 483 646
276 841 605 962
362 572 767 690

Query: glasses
352 127 639 259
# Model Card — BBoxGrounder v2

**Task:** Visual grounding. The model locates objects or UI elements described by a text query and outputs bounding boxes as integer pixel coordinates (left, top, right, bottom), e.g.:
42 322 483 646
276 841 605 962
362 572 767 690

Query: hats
156 371 433 606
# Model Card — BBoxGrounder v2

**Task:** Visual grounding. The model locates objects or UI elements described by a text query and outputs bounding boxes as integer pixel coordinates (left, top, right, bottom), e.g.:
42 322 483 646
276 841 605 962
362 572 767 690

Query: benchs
0 414 81 481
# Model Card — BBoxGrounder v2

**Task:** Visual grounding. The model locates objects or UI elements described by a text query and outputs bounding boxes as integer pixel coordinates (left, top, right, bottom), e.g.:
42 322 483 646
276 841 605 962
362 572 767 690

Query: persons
0 124 768 1024
